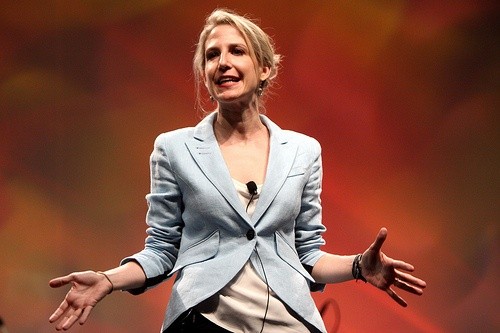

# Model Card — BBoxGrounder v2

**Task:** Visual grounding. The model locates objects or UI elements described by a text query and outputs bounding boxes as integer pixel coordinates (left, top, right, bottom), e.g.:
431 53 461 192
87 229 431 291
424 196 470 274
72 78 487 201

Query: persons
48 10 428 333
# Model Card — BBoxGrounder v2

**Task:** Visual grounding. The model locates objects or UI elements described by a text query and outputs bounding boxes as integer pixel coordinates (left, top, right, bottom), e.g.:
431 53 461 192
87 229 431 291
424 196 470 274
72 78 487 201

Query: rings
394 278 400 286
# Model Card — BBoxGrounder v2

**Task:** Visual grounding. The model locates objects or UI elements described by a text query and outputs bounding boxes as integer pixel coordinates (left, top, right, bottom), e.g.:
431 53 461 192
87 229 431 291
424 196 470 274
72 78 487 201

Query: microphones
246 180 258 195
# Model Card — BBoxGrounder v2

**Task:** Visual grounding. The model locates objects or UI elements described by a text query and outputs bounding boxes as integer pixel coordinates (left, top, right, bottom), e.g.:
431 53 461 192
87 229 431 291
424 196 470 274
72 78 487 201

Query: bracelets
96 270 114 295
350 255 367 282
